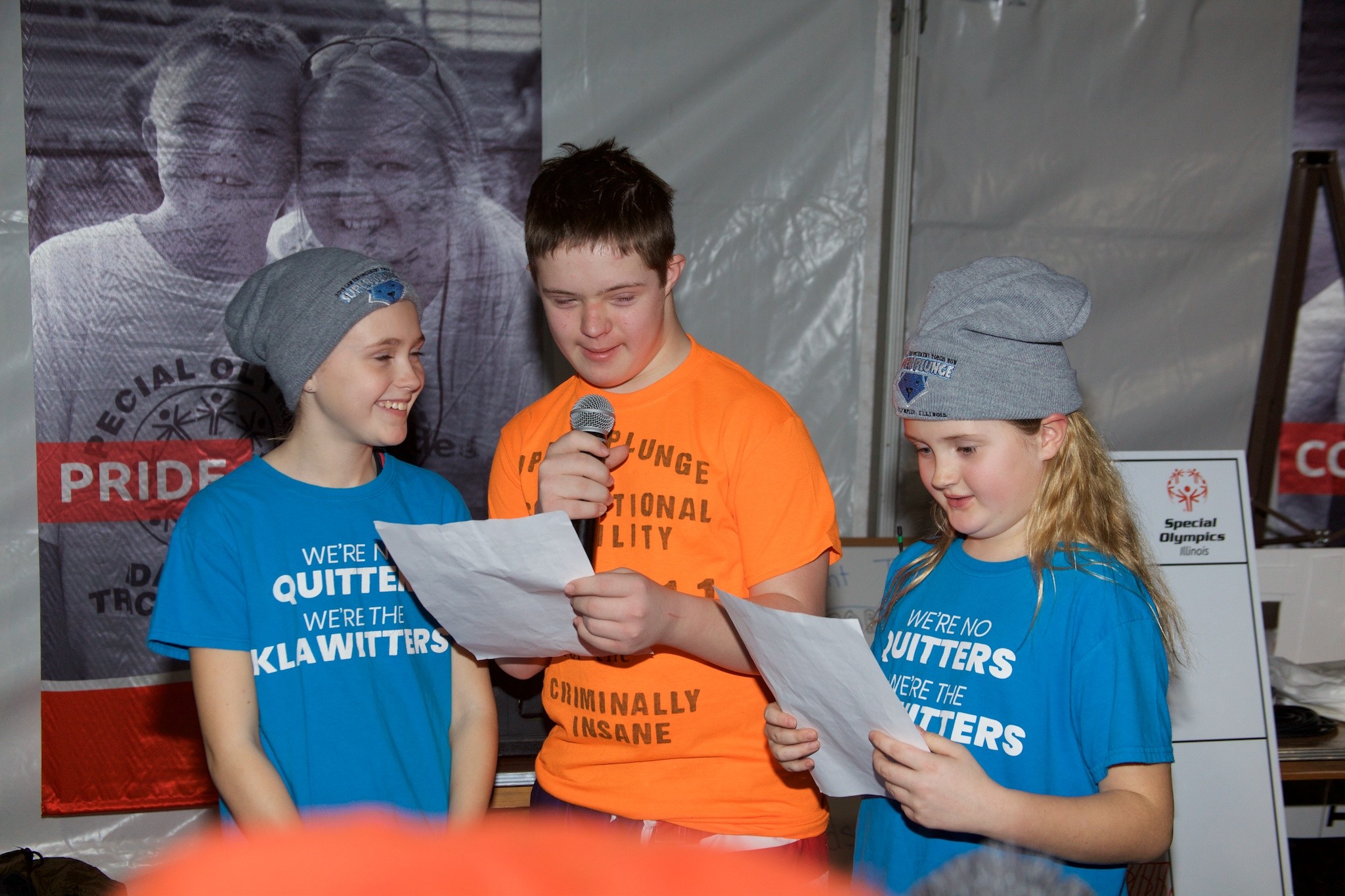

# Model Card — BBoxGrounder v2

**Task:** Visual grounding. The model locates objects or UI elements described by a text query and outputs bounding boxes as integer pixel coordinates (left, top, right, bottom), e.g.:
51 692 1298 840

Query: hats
223 246 422 411
892 255 1092 420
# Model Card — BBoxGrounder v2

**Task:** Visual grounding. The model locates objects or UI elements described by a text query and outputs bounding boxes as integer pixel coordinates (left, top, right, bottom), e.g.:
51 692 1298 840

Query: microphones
568 394 615 572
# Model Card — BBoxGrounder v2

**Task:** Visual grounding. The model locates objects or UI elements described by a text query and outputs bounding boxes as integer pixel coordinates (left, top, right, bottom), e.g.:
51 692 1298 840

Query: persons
761 258 1193 895
149 248 502 837
488 142 843 880
32 11 556 684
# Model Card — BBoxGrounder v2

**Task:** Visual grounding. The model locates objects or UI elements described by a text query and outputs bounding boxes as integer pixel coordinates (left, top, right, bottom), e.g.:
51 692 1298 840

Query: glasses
300 35 445 94
